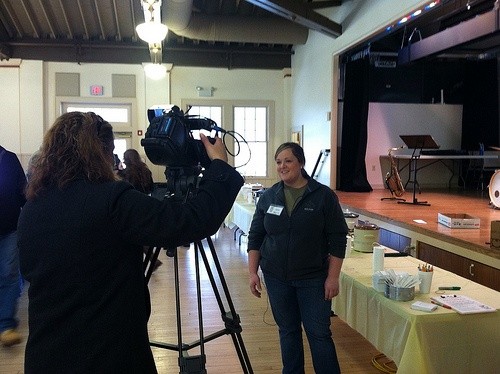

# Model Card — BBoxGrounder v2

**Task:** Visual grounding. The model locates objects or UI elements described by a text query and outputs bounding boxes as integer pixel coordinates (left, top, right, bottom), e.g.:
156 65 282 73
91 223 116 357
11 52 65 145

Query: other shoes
1 330 23 348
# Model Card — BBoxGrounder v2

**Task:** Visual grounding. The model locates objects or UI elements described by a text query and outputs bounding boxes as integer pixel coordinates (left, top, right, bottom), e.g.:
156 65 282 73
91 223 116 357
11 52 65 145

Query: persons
0 145 27 343
247 143 349 374
118 149 163 271
17 111 245 374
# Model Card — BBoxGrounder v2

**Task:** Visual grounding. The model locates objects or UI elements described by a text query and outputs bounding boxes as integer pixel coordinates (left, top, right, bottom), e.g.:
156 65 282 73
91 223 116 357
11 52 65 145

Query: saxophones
386 146 404 197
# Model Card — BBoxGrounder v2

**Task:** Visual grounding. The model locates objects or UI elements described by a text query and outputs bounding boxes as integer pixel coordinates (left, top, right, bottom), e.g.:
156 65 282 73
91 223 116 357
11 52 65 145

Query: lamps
196 85 217 97
135 0 169 80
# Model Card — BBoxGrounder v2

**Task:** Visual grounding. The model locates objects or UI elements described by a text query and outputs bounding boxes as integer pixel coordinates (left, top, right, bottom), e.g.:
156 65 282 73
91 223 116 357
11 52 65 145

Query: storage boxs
437 212 480 229
490 220 500 251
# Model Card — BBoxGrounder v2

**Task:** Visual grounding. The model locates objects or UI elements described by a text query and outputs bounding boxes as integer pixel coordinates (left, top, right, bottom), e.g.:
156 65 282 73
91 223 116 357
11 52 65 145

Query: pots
343 209 360 231
354 220 379 253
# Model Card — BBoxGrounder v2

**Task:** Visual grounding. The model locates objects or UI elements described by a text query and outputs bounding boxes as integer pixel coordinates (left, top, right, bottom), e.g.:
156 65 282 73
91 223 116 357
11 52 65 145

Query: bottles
480 142 484 155
373 246 385 273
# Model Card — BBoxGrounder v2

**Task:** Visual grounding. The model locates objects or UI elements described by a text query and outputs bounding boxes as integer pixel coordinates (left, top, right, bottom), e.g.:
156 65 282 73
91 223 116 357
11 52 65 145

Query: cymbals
490 147 500 150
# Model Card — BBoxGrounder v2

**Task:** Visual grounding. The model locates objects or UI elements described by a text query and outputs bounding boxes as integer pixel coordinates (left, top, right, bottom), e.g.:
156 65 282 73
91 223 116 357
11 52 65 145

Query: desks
221 184 500 374
380 154 498 195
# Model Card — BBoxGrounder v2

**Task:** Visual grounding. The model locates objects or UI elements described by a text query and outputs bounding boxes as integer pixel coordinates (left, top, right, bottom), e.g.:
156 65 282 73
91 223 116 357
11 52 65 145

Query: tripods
143 167 254 374
381 134 440 206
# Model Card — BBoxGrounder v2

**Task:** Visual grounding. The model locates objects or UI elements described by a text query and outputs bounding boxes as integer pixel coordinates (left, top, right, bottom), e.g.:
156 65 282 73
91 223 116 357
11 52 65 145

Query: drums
486 169 500 209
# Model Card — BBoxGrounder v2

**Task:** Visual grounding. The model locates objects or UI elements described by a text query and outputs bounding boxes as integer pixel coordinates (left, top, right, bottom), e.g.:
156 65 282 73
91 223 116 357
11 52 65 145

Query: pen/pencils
418 261 433 272
431 301 451 309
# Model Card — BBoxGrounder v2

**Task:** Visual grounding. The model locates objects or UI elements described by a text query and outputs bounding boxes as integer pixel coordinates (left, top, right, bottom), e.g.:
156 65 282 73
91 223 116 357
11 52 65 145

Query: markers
439 287 461 290
441 295 457 298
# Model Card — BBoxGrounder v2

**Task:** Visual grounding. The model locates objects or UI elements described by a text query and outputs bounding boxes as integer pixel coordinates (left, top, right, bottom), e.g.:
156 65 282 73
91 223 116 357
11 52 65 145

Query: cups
419 270 433 293
345 236 351 258
